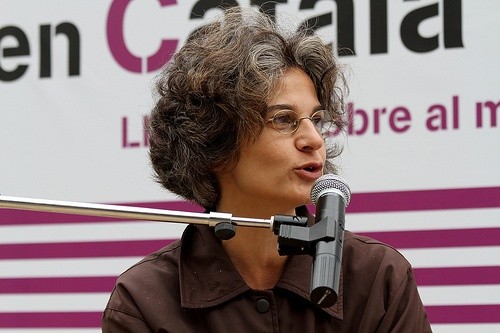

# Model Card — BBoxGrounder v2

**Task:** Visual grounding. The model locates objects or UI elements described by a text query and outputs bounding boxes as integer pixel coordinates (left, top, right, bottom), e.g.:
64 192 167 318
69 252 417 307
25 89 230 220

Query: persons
100 6 433 333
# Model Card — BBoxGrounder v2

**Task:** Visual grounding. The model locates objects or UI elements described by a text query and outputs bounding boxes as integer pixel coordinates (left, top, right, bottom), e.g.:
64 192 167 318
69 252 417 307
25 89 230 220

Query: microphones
309 174 351 308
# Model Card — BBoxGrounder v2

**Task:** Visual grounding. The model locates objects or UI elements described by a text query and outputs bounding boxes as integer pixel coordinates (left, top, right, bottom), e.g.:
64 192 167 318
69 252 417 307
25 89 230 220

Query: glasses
266 109 334 135
